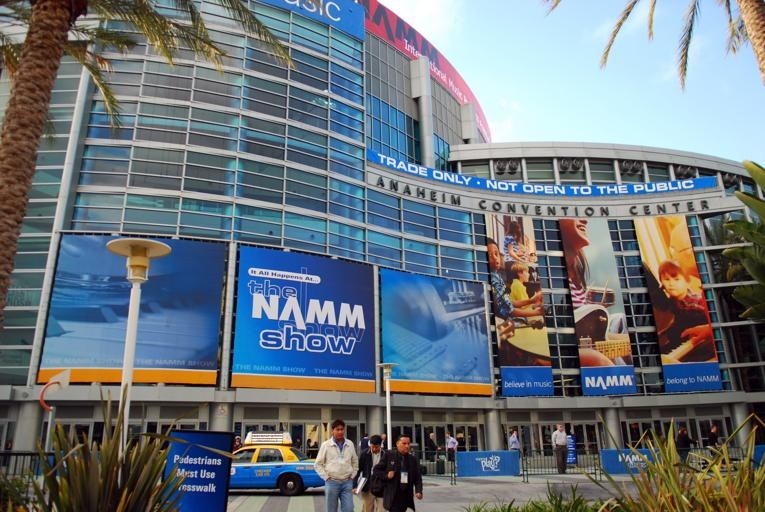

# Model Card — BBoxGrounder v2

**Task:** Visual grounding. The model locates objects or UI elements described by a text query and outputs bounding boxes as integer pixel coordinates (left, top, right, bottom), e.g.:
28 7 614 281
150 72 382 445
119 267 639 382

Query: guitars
574 303 632 360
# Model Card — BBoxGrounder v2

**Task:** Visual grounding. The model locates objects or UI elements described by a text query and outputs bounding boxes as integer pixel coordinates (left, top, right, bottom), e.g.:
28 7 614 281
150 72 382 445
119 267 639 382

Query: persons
372 434 424 512
307 438 311 457
446 431 458 459
512 263 544 322
313 419 359 512
509 429 520 461
671 223 711 345
358 432 370 451
658 260 706 326
552 423 569 474
678 428 697 463
486 237 544 327
427 432 440 461
560 219 635 367
351 435 388 512
380 434 387 450
504 220 525 262
709 424 721 457
233 434 243 450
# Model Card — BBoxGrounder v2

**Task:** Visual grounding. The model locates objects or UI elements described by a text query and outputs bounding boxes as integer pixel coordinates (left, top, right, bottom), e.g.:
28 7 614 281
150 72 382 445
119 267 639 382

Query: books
355 475 368 494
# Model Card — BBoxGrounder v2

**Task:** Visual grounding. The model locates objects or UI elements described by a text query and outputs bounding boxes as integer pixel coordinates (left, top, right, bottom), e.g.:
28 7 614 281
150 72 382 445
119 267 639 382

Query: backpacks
367 450 397 498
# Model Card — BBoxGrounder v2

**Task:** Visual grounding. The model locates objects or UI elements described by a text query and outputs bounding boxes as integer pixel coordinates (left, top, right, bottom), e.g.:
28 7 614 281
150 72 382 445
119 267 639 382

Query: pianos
641 261 716 362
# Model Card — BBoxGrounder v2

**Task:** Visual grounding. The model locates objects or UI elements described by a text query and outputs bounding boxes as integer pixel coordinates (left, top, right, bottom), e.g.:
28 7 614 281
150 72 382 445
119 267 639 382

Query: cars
229 431 325 496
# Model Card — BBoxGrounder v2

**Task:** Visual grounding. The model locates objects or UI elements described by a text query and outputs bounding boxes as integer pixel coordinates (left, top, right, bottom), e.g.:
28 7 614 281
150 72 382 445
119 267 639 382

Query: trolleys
696 441 744 472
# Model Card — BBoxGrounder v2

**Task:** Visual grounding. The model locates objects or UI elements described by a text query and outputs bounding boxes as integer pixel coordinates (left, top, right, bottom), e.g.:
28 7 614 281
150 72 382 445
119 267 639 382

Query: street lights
106 238 171 488
376 363 400 450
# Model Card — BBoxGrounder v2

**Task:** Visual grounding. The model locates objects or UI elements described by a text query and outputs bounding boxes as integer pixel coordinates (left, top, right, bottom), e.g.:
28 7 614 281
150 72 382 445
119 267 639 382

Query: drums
590 287 616 306
501 325 551 366
